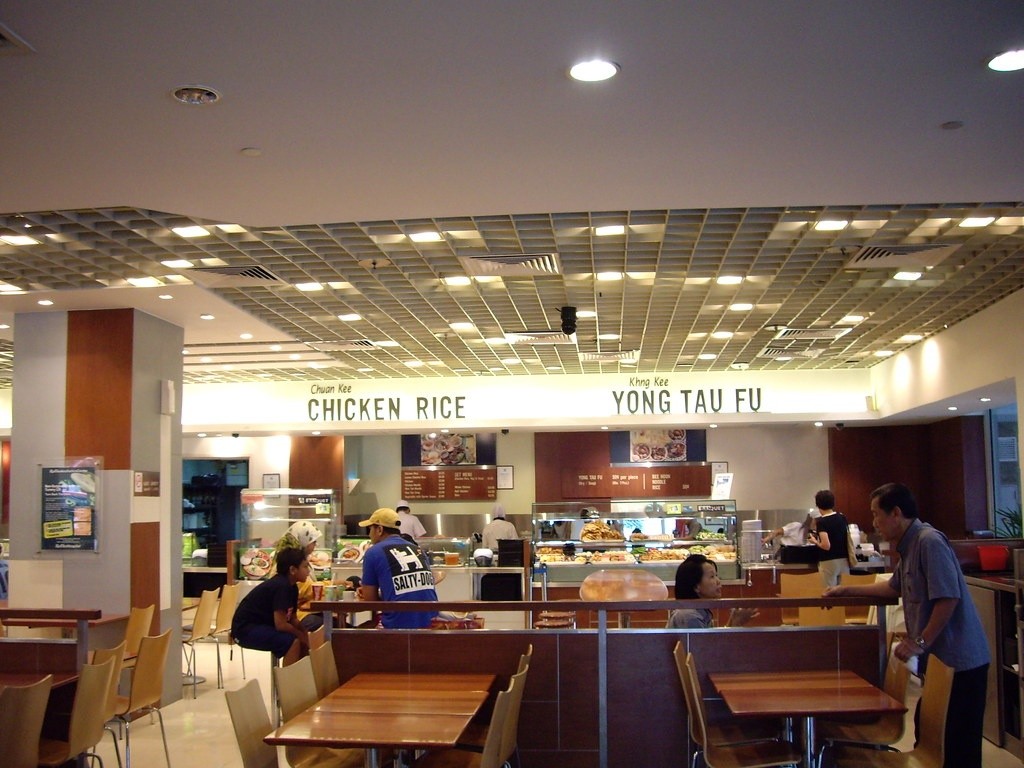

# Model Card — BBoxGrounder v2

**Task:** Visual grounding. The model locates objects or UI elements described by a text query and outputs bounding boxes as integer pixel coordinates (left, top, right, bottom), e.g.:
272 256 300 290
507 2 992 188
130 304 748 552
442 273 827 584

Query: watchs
914 635 927 650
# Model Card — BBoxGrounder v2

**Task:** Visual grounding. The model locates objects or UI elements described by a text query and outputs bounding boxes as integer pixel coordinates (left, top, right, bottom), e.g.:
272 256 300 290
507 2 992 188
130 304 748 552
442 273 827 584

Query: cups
312 585 322 601
343 591 354 602
325 586 337 601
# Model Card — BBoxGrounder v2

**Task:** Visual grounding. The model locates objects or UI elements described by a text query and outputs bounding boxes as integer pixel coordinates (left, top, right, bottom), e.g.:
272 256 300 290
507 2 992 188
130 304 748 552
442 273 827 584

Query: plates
243 565 265 576
338 546 363 563
359 540 373 552
252 557 268 568
240 556 251 565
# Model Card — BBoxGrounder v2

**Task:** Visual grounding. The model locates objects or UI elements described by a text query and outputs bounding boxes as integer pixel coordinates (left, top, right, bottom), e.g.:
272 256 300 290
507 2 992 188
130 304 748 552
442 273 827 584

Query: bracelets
723 625 727 627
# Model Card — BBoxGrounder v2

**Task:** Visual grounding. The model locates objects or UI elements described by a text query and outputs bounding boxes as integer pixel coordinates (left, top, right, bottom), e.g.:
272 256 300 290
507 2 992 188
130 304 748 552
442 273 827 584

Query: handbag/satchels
841 513 858 569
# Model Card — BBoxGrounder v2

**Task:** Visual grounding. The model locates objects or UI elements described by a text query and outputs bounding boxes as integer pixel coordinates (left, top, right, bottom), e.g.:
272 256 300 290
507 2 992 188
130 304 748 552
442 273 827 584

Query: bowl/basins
445 553 459 564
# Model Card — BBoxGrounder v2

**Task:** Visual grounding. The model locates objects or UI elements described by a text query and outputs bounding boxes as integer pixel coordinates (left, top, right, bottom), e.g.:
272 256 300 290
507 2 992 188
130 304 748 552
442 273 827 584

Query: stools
539 613 576 629
533 621 576 629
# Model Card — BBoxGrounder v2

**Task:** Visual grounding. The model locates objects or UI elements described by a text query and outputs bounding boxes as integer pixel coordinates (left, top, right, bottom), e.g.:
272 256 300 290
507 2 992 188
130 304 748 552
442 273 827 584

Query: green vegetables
630 547 647 554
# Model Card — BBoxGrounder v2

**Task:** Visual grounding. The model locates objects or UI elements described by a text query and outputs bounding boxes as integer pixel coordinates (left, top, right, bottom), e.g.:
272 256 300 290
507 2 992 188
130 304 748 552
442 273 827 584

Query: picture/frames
262 474 282 498
495 465 514 489
711 461 728 486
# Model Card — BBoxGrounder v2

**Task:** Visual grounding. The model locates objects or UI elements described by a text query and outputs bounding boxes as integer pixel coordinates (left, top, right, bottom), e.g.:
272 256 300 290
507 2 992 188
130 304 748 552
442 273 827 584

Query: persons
542 521 551 530
230 548 311 668
664 554 761 630
631 528 646 549
270 521 357 632
549 521 568 540
482 517 519 555
0 544 8 601
675 519 703 541
821 479 992 768
396 500 427 540
808 489 849 593
606 520 623 534
761 507 823 562
355 508 439 630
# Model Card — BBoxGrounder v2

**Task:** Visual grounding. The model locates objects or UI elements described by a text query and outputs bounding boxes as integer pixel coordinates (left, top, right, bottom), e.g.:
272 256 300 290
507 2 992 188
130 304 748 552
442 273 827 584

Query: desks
0 672 80 690
182 598 206 685
261 710 474 768
720 686 909 768
2 612 129 639
707 670 873 768
340 672 497 763
300 590 382 629
305 689 488 768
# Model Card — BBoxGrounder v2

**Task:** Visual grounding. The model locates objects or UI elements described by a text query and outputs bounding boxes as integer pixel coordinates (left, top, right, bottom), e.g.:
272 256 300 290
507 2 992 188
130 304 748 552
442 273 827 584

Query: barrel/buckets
977 544 1008 570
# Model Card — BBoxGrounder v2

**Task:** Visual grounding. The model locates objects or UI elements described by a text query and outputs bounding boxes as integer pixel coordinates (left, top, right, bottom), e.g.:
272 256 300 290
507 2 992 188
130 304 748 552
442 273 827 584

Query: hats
358 509 401 530
396 500 410 512
493 503 505 520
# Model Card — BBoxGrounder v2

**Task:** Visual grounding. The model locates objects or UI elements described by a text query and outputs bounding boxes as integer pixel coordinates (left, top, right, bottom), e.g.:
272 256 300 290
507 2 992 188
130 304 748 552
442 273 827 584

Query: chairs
0 571 957 768
